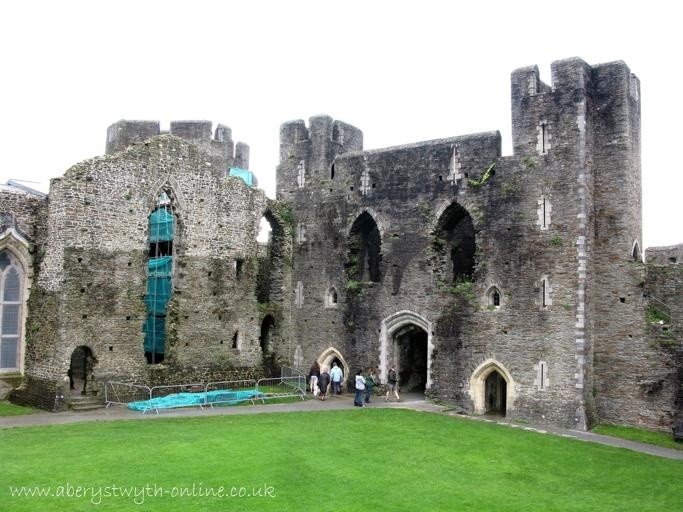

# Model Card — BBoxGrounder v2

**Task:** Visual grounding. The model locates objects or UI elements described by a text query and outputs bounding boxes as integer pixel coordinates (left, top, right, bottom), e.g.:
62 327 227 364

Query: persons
363 368 379 403
308 360 319 396
384 364 401 402
329 361 344 395
317 367 330 400
352 369 366 407
